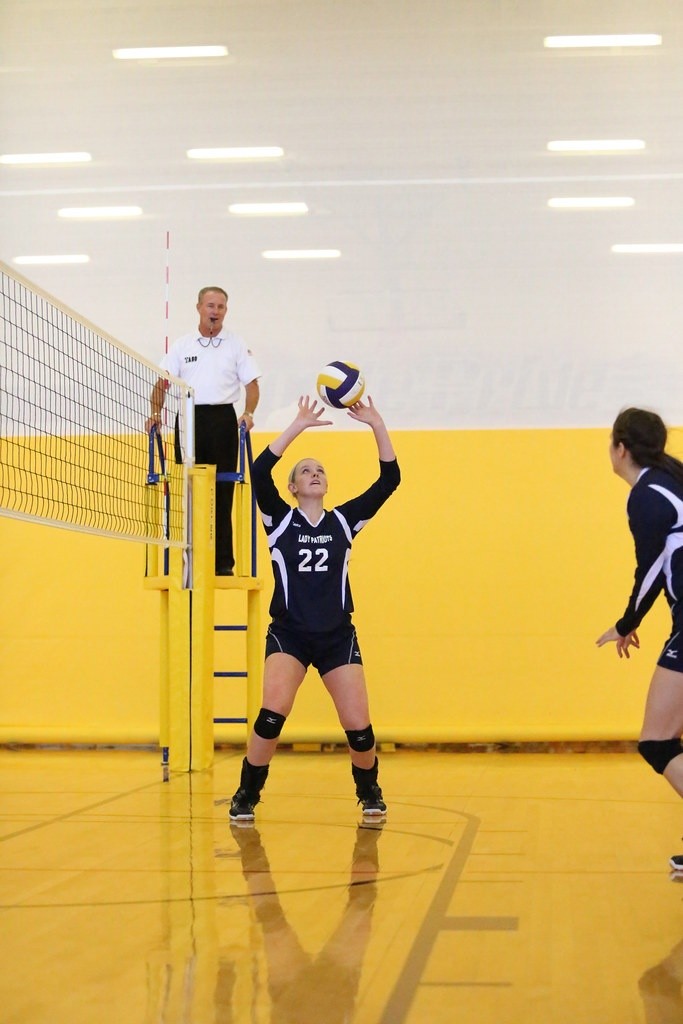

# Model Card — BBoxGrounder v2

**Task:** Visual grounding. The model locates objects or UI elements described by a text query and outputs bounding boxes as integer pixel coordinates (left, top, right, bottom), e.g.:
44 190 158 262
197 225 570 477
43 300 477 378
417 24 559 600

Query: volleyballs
316 359 368 408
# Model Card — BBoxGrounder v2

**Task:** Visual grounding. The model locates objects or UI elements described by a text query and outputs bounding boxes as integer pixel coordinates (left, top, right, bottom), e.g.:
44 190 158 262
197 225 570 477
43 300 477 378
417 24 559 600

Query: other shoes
669 854 683 871
215 567 234 576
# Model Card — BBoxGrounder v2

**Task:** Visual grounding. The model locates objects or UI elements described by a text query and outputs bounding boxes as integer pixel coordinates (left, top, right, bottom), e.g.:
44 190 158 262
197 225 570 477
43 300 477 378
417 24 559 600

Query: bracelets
244 411 254 419
152 413 161 416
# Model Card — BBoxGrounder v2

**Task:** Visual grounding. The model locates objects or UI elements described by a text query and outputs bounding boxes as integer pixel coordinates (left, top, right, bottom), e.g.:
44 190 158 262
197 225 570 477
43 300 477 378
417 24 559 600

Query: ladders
145 421 265 783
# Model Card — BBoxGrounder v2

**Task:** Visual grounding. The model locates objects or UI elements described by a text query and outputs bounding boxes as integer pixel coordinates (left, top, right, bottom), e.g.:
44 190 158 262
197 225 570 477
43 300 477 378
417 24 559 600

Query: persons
225 820 383 1023
146 286 260 577
228 395 401 826
597 407 683 870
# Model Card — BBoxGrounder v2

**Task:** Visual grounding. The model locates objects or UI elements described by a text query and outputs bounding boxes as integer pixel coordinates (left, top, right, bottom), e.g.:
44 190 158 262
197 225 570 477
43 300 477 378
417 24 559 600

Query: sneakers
359 798 387 815
229 794 256 821
